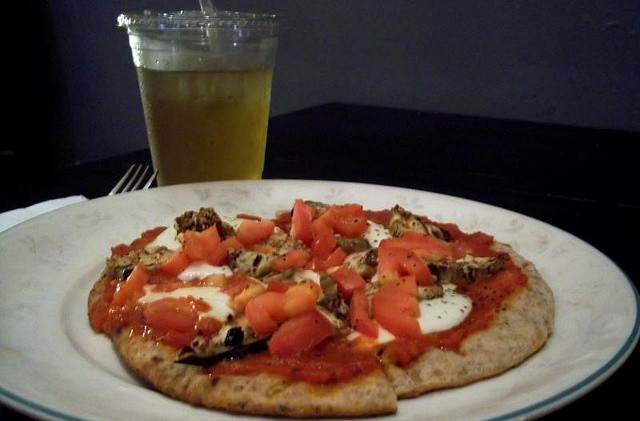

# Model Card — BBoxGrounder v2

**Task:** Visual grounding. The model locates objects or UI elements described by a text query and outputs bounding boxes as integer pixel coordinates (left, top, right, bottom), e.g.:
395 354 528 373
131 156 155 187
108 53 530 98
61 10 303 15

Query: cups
115 12 278 187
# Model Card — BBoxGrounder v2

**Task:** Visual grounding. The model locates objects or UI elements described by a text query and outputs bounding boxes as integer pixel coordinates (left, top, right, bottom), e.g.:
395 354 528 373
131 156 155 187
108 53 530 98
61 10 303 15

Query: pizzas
87 199 556 420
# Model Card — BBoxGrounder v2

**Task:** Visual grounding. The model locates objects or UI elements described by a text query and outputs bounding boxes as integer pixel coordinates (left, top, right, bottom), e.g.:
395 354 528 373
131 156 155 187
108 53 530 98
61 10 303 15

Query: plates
1 179 640 419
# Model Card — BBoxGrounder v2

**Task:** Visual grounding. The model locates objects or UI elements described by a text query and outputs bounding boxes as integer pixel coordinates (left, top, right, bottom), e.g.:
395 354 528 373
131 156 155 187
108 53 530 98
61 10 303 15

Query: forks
88 163 159 195
